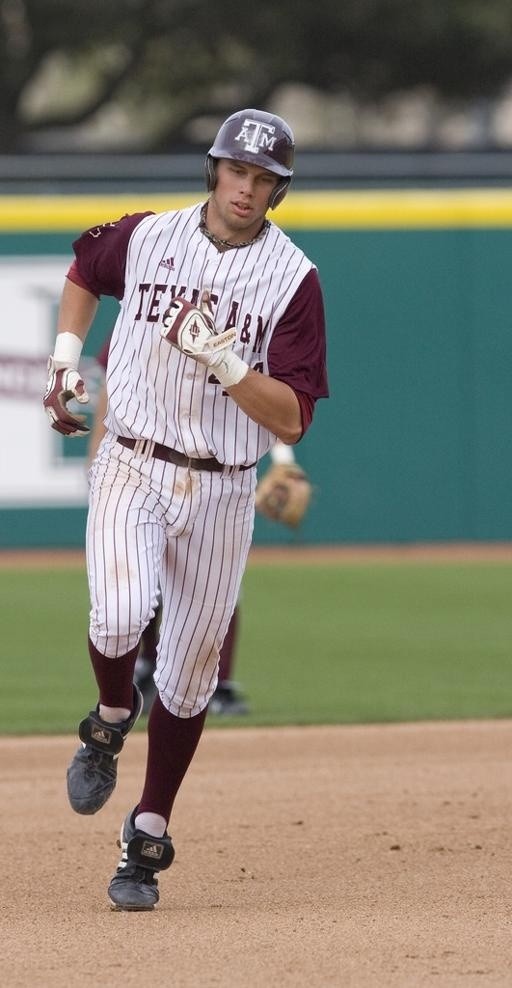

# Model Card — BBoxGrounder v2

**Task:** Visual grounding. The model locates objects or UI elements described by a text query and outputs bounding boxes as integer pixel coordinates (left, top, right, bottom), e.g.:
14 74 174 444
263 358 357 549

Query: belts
117 435 259 473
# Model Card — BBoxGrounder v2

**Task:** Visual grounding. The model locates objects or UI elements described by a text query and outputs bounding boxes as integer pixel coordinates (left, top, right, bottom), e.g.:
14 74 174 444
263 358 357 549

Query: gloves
157 289 239 370
42 354 92 438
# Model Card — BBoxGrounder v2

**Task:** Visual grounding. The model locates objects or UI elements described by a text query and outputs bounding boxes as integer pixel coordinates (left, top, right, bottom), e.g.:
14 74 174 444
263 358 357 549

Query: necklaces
202 208 270 248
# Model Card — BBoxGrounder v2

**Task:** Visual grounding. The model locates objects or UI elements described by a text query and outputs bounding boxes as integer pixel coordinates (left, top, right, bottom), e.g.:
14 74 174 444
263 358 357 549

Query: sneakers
133 662 160 716
208 681 252 718
105 802 177 915
66 681 144 816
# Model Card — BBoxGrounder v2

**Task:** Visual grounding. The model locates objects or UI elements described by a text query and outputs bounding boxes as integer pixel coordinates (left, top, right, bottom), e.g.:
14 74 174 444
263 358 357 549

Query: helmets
202 107 296 211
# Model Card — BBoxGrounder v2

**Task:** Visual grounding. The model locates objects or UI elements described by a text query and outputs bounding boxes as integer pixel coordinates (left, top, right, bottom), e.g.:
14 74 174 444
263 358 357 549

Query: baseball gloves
253 461 314 528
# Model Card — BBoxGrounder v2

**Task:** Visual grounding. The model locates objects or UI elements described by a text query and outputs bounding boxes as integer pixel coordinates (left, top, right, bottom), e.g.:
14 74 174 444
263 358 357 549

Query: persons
43 110 329 911
124 440 312 715
